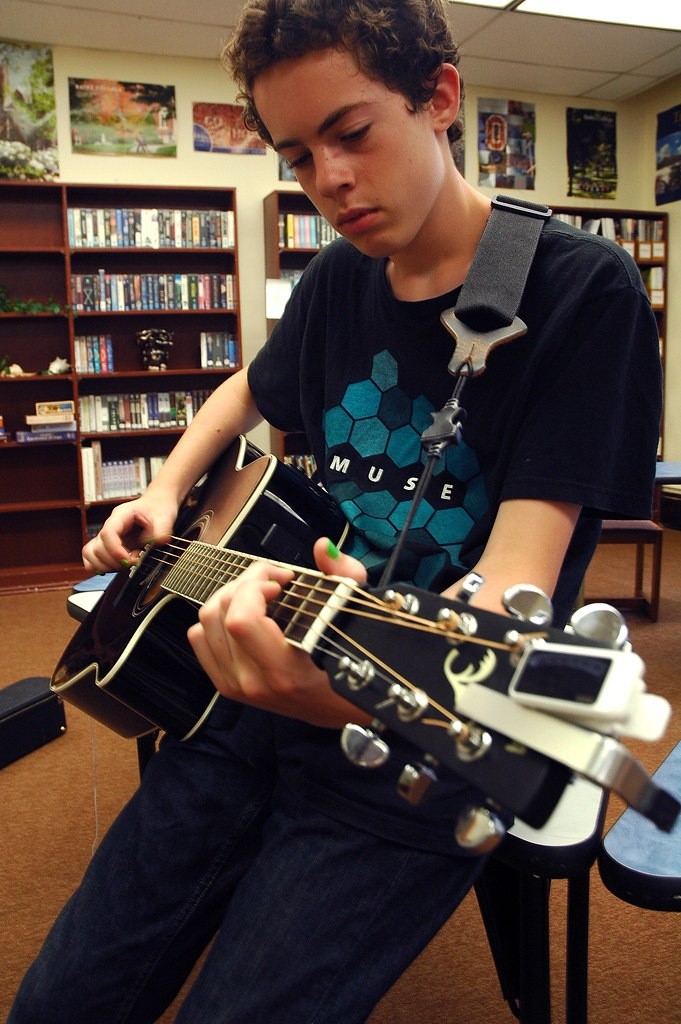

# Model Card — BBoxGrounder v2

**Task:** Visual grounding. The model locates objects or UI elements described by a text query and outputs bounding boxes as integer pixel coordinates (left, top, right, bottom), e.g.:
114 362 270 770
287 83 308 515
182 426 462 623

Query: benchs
583 520 665 622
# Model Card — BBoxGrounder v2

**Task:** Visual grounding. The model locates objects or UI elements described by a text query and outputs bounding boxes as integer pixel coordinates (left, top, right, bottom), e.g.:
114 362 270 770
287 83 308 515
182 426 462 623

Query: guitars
49 434 681 881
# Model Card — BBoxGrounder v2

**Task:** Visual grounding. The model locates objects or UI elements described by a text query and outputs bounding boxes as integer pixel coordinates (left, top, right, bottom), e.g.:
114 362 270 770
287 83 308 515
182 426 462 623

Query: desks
67 592 607 1024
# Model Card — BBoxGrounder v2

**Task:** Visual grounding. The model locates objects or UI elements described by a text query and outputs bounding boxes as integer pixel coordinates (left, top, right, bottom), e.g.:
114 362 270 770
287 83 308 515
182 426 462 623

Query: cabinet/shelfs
1 178 243 583
257 190 668 461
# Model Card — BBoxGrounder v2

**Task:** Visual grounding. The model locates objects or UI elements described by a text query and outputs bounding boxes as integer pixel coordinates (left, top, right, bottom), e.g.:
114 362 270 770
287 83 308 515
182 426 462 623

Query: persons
5 0 664 1024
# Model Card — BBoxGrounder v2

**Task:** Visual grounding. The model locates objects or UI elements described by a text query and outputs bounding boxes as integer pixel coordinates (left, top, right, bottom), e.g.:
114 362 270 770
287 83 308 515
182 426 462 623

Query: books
200 332 236 368
81 441 167 503
552 214 664 306
70 268 233 312
277 214 344 249
279 269 306 290
16 400 77 443
67 207 235 249
74 333 114 375
78 389 215 432
